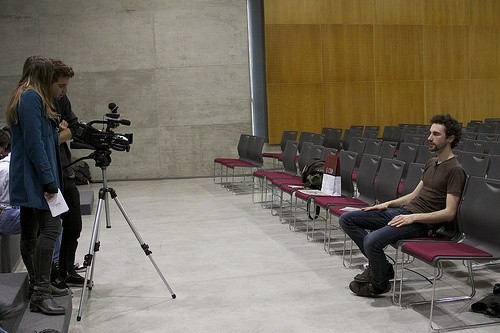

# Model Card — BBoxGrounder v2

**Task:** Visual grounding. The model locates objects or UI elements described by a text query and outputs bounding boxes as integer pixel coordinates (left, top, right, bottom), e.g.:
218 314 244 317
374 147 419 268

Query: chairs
213 116 500 333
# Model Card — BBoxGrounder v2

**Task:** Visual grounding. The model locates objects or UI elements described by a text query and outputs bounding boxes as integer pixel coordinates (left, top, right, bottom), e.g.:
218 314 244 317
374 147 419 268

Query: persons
339 114 467 296
0 126 87 272
46 58 129 296
6 55 70 314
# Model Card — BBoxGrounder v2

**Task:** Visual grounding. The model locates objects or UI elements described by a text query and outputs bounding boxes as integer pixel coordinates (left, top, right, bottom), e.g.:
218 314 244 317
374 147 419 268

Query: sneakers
57 263 94 288
49 271 74 299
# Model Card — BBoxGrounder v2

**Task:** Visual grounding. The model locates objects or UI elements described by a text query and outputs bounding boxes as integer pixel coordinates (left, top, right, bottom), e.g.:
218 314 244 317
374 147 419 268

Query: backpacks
301 157 327 191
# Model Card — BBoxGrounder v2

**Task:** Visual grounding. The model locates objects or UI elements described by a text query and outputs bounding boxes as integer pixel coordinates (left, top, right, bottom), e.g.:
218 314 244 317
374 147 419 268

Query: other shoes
353 261 395 283
349 280 391 297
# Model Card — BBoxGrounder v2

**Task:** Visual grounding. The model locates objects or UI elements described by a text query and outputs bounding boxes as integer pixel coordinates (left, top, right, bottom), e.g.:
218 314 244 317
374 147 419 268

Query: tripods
77 150 175 321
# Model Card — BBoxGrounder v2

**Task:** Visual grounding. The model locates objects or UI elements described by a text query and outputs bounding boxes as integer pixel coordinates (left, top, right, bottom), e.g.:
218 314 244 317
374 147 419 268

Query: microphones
113 119 130 126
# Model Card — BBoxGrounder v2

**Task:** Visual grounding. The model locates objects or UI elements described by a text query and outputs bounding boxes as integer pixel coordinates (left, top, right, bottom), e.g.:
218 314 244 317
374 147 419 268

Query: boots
29 247 66 315
19 235 69 298
